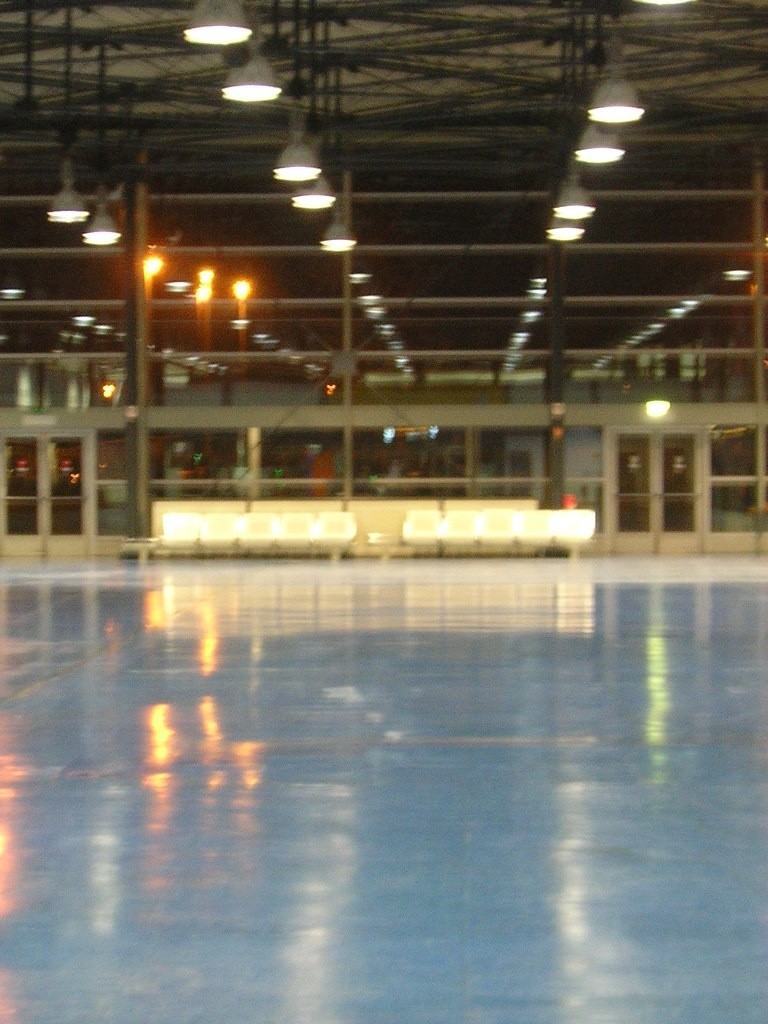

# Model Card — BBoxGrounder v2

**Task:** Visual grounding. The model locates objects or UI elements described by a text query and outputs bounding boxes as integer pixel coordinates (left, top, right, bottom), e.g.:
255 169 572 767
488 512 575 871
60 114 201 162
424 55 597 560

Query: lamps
44 1 646 256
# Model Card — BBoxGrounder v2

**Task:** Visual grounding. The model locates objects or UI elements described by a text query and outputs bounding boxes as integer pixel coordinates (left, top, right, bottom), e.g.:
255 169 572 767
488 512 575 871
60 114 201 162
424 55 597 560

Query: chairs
156 508 598 561
